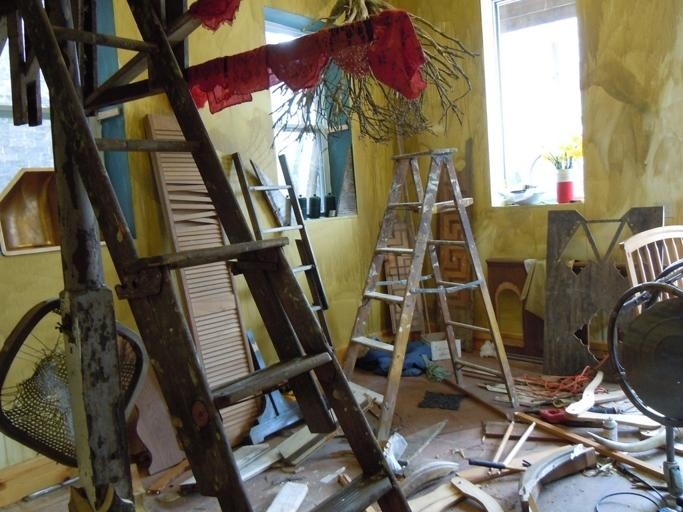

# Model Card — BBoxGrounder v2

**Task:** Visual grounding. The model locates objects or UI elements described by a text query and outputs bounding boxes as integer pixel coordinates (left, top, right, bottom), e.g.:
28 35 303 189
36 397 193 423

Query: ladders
230 151 336 355
341 147 520 454
23 0 412 512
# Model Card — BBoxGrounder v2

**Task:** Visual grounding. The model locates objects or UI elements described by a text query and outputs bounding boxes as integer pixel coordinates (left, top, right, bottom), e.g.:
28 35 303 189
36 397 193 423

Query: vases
554 167 576 204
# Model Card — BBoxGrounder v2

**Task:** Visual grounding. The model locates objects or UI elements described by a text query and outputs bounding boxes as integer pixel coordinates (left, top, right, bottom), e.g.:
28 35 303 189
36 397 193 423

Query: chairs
617 223 683 323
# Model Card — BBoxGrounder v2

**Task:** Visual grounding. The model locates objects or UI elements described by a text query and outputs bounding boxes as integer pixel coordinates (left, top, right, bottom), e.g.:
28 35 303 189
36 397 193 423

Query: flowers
541 135 583 171
